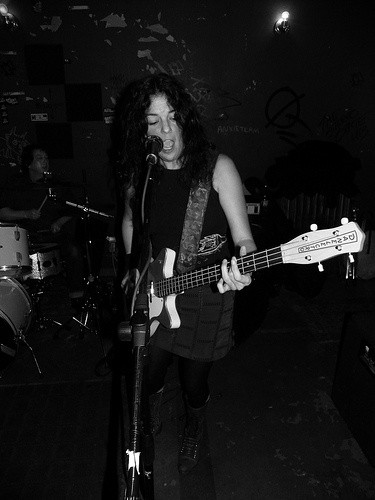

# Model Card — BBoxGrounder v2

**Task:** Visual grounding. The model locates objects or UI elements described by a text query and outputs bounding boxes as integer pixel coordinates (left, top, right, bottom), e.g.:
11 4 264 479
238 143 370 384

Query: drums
25 252 58 283
0 222 31 275
0 278 32 355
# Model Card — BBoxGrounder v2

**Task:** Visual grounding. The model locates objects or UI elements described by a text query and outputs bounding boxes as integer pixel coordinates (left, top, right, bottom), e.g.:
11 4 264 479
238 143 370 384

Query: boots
178 394 210 474
148 384 165 438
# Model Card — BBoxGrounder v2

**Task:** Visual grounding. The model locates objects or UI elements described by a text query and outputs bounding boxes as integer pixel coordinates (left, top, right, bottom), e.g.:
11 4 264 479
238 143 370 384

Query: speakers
331 310 375 473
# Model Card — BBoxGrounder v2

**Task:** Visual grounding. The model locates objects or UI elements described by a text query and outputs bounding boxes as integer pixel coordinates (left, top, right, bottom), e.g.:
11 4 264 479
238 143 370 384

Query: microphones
145 135 164 166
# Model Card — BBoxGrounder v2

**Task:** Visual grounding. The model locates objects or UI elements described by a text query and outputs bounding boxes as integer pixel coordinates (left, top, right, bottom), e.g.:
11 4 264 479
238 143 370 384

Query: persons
0 145 107 319
110 73 256 500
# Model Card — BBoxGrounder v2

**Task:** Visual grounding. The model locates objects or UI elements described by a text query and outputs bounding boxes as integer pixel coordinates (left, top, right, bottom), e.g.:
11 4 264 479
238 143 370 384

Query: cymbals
3 182 89 189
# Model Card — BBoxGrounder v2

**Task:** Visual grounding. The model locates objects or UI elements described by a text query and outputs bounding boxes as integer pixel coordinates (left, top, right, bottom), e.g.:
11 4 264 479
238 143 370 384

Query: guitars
133 215 366 348
